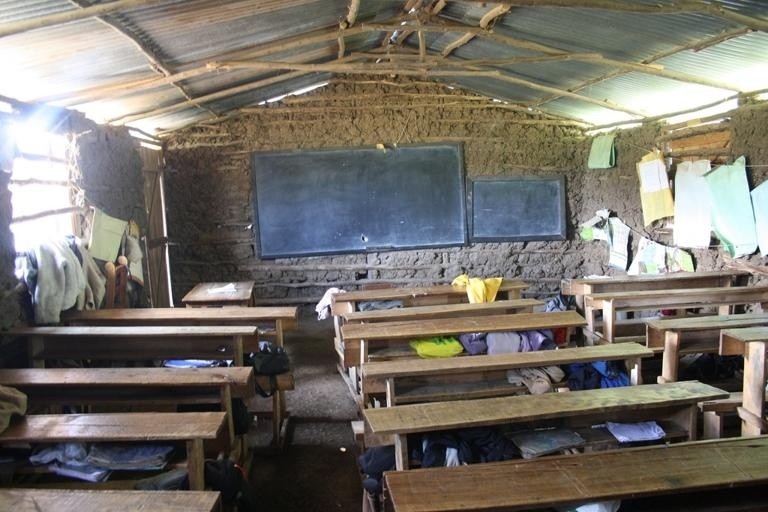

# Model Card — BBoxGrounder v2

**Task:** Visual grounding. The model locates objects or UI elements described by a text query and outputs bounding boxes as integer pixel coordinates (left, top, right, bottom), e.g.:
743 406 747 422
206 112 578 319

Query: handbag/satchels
247 344 291 376
357 301 403 311
409 335 464 358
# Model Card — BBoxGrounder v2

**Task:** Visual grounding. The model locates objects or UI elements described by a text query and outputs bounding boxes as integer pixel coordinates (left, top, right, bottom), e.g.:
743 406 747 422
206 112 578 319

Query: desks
329 269 768 511
0 308 301 512
181 282 258 308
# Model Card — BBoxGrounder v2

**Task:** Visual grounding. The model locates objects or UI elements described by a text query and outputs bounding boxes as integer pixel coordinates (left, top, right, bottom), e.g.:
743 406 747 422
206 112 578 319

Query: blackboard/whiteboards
251 141 470 260
465 175 567 241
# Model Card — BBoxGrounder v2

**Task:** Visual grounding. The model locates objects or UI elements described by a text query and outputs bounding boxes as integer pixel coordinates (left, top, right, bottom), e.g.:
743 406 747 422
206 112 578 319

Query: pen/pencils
591 423 606 429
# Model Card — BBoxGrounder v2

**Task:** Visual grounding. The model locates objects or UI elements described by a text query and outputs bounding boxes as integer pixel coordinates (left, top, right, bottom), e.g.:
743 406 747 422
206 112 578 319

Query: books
605 417 667 444
85 440 176 472
47 461 113 483
164 357 226 368
512 426 589 460
